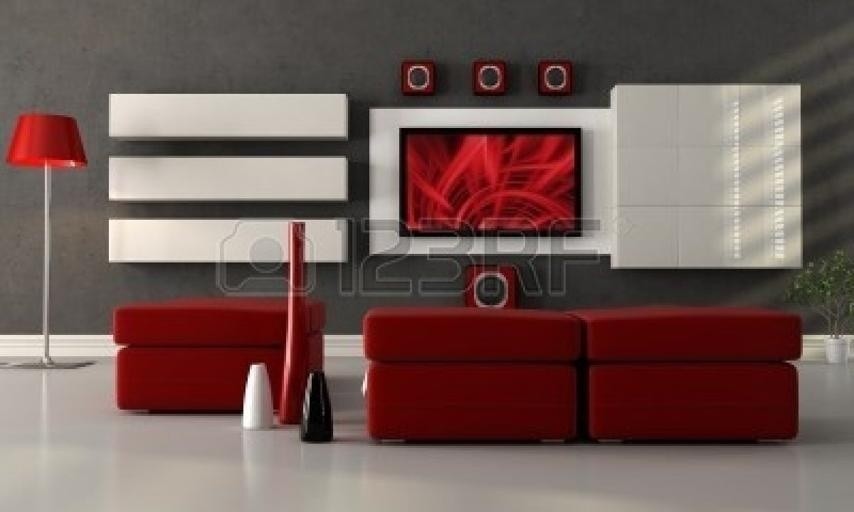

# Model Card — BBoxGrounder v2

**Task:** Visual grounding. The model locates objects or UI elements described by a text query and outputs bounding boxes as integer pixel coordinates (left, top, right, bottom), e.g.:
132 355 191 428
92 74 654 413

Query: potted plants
782 249 854 364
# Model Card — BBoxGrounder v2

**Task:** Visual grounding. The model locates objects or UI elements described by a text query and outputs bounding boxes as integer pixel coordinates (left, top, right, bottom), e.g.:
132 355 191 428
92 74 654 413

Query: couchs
363 308 579 443
568 308 804 443
113 302 325 414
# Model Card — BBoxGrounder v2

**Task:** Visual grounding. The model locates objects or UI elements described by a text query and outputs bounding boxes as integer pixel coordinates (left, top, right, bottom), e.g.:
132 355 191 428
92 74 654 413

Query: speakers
465 262 517 311
537 58 572 97
401 59 435 96
471 59 504 98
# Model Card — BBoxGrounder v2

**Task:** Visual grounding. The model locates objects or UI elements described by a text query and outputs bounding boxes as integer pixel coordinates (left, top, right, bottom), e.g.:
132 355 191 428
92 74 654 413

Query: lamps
0 114 94 370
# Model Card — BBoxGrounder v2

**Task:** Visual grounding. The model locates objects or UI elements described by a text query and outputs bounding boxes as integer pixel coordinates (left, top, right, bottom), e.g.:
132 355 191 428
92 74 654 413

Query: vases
241 363 273 428
279 222 304 425
300 370 333 441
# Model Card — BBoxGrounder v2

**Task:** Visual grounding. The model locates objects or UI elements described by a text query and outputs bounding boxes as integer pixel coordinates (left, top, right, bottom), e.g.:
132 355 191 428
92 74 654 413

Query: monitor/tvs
398 126 584 239
